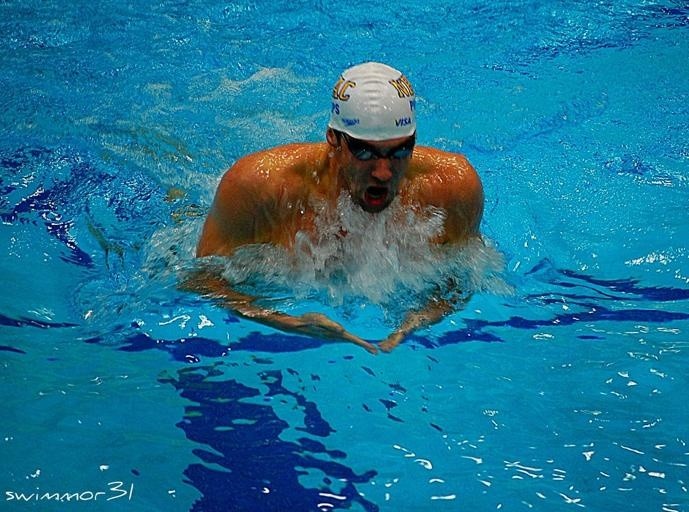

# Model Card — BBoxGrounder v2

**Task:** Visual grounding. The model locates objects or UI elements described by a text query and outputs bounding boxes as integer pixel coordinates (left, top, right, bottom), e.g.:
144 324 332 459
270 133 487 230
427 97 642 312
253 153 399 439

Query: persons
176 63 485 355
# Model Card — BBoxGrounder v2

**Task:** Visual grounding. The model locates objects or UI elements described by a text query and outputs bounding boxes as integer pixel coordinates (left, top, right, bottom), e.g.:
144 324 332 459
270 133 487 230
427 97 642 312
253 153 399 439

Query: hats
327 60 416 142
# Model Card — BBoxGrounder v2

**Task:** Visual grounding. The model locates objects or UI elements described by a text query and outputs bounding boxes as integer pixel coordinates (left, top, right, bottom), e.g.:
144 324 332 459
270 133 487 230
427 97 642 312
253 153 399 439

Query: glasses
342 131 415 162
